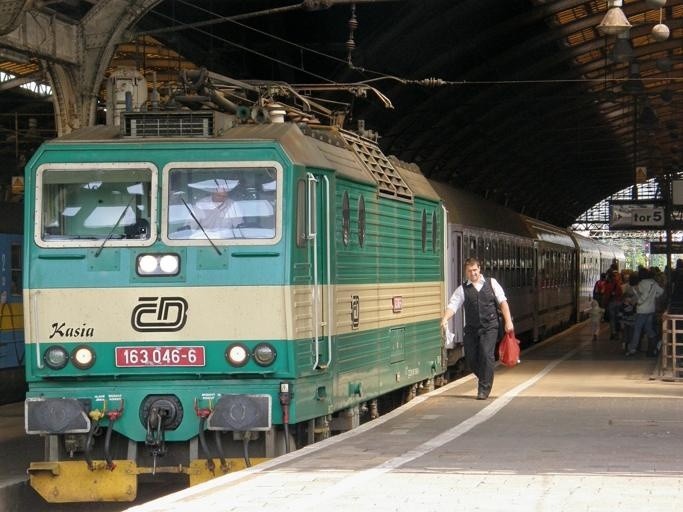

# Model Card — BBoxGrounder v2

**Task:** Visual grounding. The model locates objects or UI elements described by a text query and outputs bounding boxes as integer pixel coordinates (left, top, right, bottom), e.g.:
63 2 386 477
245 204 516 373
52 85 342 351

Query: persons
440 257 514 399
174 182 244 241
587 257 682 361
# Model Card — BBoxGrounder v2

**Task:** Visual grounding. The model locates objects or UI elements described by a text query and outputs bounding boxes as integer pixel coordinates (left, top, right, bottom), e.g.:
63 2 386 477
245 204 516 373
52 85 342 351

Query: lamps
593 1 634 37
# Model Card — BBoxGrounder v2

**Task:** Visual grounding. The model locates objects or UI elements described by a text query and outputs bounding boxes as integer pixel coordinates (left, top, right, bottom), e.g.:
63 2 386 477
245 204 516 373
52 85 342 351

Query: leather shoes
477 390 490 400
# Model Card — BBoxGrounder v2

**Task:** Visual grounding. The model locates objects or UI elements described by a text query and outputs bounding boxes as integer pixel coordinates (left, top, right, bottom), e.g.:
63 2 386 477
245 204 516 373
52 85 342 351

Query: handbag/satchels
497 331 522 368
497 309 514 341
